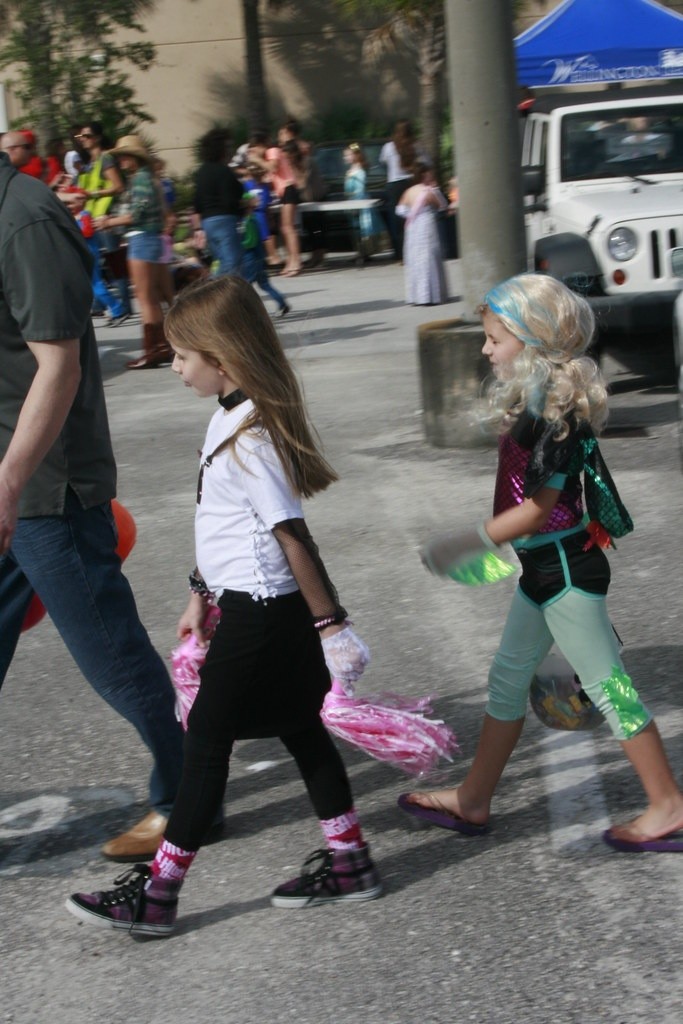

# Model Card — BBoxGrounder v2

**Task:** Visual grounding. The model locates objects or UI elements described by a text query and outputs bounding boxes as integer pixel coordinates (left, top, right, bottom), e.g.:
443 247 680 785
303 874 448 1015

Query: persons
378 117 449 305
0 120 174 370
64 272 383 938
342 140 388 266
0 149 185 862
188 118 328 316
397 271 682 852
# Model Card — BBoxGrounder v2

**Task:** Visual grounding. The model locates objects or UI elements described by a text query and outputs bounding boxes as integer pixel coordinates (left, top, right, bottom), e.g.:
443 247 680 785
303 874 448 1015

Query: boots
124 322 174 370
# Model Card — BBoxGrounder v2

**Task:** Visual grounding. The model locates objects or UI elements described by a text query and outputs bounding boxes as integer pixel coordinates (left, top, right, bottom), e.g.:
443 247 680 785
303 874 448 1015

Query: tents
516 0 683 94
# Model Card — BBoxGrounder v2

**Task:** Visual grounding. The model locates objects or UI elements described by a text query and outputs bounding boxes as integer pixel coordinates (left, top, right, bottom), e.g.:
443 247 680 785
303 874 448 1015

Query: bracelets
476 516 500 552
194 228 204 233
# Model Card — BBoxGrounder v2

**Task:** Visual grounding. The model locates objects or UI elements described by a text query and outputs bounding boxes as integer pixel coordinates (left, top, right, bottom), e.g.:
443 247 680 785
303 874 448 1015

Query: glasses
74 134 95 139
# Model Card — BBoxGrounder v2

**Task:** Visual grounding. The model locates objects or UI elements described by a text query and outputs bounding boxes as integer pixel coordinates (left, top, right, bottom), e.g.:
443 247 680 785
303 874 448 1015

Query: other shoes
107 312 131 328
274 305 291 318
263 253 305 278
88 308 105 316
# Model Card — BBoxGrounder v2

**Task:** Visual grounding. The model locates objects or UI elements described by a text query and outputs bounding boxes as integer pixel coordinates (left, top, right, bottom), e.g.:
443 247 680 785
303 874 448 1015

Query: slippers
397 792 475 837
604 817 683 852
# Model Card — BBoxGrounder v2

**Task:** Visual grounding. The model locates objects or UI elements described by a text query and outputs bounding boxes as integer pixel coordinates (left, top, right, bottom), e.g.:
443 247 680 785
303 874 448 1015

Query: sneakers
66 864 187 936
271 848 384 908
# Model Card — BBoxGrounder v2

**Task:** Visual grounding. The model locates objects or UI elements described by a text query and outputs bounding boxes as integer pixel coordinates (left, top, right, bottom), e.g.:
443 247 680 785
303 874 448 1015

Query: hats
104 135 155 161
0 129 37 149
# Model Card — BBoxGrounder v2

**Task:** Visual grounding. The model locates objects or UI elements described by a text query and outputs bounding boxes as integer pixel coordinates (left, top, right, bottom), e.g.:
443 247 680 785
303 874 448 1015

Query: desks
270 199 382 263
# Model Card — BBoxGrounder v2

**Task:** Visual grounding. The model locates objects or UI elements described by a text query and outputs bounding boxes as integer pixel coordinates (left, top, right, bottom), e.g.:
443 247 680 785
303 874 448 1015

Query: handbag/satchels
237 217 257 248
525 626 606 731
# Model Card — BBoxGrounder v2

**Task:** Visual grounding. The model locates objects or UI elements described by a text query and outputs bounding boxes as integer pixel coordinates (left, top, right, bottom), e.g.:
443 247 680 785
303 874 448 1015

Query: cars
311 140 388 213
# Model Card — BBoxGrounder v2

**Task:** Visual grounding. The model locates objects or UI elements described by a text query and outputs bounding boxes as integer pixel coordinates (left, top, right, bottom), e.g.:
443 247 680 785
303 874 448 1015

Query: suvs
519 77 682 374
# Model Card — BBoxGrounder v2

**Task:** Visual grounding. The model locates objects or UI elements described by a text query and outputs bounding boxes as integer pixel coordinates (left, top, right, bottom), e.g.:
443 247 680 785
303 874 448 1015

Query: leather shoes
103 802 226 860
